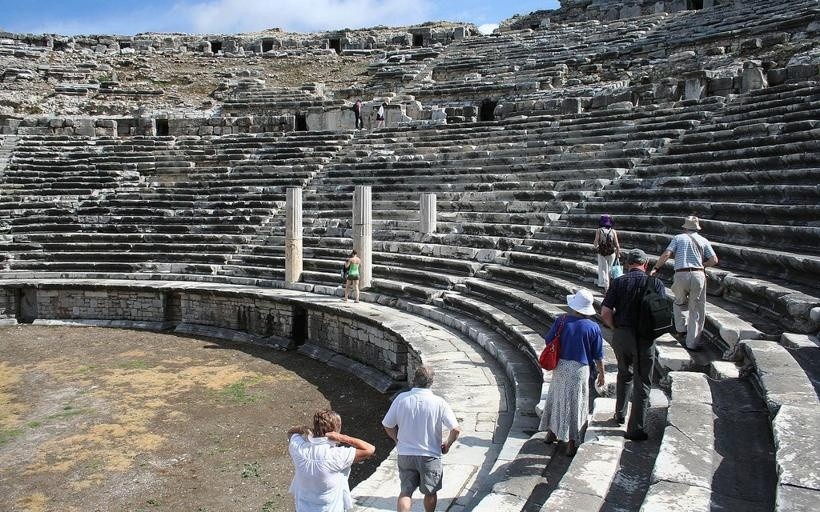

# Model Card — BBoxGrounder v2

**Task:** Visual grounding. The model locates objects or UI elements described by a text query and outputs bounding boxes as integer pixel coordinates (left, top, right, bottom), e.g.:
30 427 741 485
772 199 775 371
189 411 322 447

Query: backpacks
353 102 358 112
633 275 673 345
600 227 615 257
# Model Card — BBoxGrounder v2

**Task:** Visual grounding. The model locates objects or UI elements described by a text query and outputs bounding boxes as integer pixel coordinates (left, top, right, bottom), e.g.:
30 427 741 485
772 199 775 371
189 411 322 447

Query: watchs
652 266 660 271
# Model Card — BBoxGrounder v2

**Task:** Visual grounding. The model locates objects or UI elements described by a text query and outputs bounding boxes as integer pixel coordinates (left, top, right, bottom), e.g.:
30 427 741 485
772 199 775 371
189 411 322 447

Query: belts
675 268 703 271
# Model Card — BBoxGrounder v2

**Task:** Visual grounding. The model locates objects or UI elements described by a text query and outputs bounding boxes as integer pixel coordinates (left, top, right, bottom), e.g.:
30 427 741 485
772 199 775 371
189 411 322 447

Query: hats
567 289 595 316
627 248 649 260
681 215 700 231
602 216 611 227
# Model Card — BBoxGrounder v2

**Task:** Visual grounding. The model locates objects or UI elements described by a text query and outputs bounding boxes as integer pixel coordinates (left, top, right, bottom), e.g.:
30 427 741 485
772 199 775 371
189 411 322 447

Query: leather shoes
625 433 647 440
614 414 624 424
567 447 577 454
544 435 556 442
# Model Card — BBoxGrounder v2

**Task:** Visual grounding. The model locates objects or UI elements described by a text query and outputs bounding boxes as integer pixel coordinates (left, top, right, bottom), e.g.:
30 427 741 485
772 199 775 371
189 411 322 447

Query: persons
601 249 666 442
593 216 620 295
650 216 718 351
355 99 363 129
539 290 605 457
342 250 361 302
287 411 375 512
376 103 387 127
382 367 460 512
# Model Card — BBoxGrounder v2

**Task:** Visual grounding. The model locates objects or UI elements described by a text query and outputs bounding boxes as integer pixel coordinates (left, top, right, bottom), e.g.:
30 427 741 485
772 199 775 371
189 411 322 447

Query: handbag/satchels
540 316 564 370
